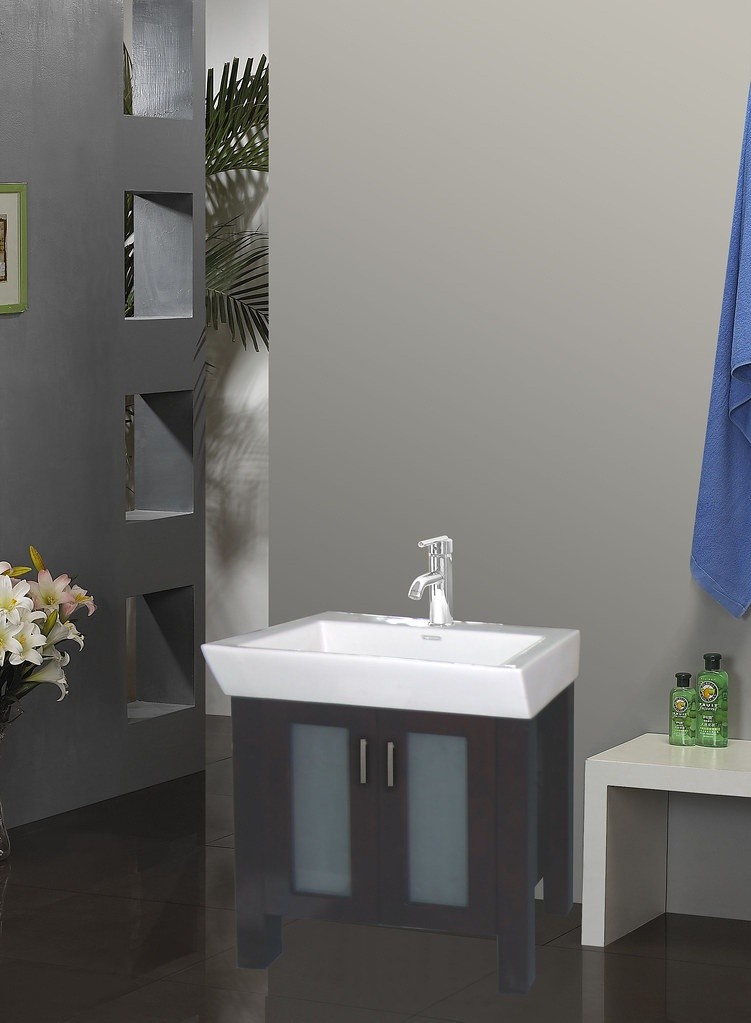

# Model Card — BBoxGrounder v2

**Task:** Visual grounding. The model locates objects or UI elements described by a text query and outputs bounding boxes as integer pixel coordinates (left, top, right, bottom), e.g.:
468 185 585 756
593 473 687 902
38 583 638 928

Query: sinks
200 611 580 719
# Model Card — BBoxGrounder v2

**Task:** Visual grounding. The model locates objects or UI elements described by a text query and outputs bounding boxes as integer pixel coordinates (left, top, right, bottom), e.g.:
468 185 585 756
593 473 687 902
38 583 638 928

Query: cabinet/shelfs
255 697 498 936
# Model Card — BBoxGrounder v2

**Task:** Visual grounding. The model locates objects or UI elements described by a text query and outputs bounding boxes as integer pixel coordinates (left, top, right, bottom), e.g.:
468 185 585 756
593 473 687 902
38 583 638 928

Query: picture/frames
0 181 28 314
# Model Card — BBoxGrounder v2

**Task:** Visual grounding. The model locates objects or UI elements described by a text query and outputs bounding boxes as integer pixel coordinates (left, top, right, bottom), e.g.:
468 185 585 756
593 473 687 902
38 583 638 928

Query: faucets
408 535 453 628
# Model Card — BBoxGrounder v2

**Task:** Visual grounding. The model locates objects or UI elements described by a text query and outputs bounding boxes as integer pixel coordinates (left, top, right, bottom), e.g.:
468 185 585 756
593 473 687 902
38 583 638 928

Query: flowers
0 543 99 744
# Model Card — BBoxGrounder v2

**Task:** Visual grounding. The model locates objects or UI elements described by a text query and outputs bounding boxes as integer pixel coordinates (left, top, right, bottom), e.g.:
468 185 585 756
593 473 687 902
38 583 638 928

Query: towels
690 82 751 620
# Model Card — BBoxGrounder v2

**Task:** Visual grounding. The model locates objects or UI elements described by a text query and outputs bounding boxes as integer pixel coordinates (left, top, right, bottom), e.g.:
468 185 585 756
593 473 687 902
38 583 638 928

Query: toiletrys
696 653 728 747
669 672 696 746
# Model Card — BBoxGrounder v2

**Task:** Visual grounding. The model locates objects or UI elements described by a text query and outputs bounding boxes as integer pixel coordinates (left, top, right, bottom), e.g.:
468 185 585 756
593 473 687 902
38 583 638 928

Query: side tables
581 732 751 947
